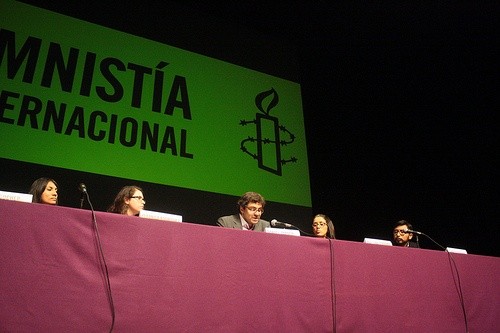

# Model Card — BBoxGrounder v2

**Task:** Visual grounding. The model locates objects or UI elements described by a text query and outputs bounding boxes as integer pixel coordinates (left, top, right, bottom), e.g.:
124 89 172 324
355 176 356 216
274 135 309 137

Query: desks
0 196 500 332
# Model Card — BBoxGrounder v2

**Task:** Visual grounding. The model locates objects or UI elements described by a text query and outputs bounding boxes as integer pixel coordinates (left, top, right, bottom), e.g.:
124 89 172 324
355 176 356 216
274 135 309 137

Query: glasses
244 206 264 214
129 196 145 201
312 222 328 228
393 230 406 236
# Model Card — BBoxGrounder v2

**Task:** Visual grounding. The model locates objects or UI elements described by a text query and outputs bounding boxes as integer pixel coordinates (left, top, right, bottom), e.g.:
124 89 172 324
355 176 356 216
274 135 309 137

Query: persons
28 177 59 206
312 214 336 239
393 220 418 248
107 186 146 216
216 191 271 232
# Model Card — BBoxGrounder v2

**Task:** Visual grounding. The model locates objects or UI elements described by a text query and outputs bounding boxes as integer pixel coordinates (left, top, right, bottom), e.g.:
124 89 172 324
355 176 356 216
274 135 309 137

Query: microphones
79 183 86 193
403 228 421 235
271 219 291 227
